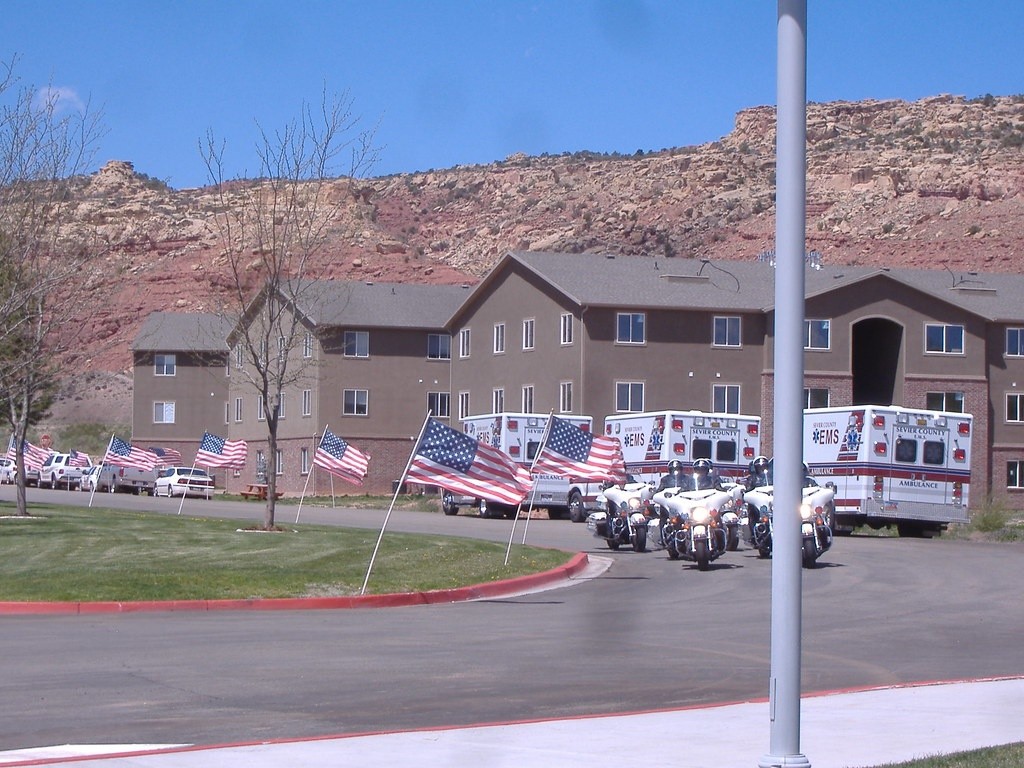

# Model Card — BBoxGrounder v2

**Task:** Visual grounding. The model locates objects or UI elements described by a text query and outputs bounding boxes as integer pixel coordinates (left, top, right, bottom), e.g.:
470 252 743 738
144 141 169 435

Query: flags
148 447 183 465
313 429 372 487
104 436 157 472
194 432 248 470
532 415 629 489
70 449 88 465
402 417 534 505
7 434 50 470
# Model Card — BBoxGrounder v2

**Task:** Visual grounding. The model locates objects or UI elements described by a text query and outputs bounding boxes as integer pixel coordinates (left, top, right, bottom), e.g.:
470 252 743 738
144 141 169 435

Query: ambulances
803 404 973 538
567 409 762 522
441 412 594 519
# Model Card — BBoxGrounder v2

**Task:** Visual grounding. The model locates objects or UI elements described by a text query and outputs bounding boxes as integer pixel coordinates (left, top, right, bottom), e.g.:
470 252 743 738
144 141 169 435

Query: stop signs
40 434 52 449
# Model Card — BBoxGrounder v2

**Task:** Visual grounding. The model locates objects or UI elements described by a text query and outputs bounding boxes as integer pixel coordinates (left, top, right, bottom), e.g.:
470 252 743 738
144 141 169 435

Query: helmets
667 459 683 469
692 458 710 467
802 461 809 473
706 459 713 470
749 456 773 468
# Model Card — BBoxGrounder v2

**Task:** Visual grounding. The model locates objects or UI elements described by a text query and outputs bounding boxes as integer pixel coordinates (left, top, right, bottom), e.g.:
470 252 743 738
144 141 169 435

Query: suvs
37 454 92 490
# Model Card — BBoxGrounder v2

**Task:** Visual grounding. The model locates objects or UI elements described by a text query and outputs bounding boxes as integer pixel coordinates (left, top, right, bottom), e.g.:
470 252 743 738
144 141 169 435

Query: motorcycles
588 470 657 552
668 470 738 571
800 472 837 569
742 465 774 559
648 487 682 559
709 468 747 551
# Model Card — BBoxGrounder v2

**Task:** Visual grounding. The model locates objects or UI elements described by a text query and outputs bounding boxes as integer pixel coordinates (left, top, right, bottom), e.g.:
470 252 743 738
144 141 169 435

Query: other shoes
750 535 756 544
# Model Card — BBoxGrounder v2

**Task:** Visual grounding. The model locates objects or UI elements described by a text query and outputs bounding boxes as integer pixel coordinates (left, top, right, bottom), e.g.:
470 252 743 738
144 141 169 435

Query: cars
79 465 102 492
153 467 215 500
14 469 38 487
97 461 158 497
0 459 5 480
4 460 17 485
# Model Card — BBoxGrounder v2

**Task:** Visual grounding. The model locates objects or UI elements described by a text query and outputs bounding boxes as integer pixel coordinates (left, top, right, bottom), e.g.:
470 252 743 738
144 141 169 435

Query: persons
656 459 690 546
705 456 830 549
603 461 638 536
675 458 728 556
